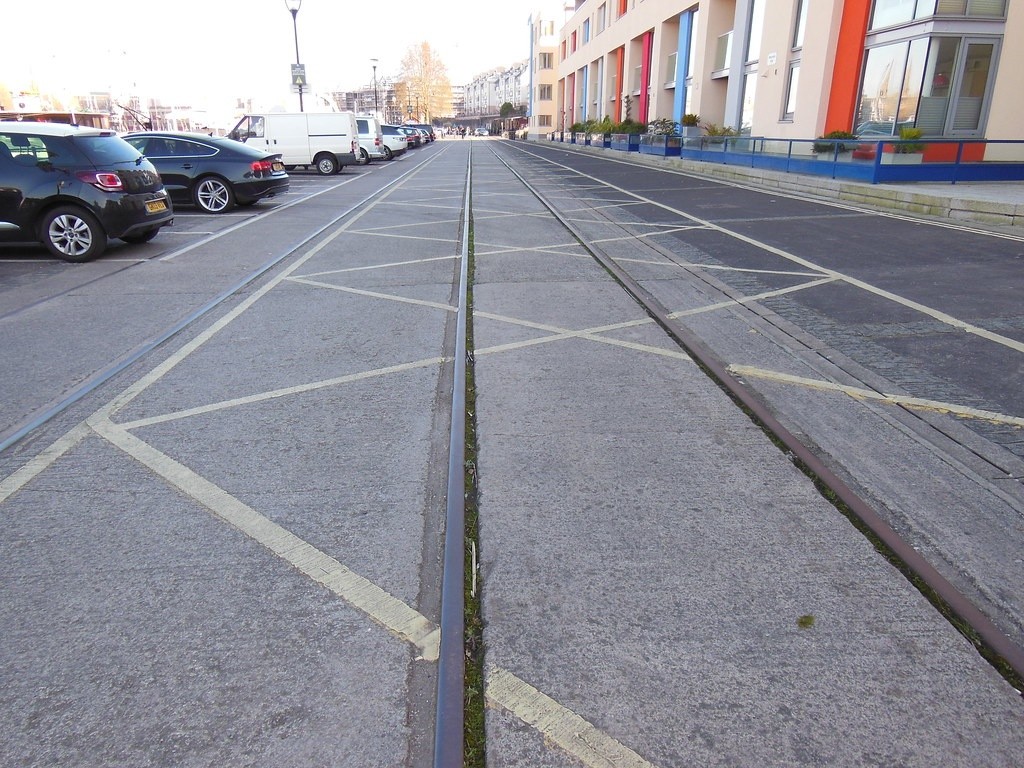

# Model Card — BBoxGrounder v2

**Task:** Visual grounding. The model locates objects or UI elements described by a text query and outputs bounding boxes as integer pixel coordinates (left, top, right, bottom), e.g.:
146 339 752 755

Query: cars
382 124 435 161
0 117 174 263
120 130 290 214
473 128 490 138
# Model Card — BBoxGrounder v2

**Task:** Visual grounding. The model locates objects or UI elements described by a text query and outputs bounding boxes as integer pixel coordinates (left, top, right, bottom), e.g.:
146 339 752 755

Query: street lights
407 85 413 119
283 0 303 113
370 58 380 116
415 92 420 121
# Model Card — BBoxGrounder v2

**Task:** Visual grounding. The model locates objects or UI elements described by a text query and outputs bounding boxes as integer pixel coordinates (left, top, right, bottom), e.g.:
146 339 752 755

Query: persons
453 127 466 139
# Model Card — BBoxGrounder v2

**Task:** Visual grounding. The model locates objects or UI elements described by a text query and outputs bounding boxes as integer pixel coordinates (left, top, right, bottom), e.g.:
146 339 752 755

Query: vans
356 115 387 166
220 111 362 177
